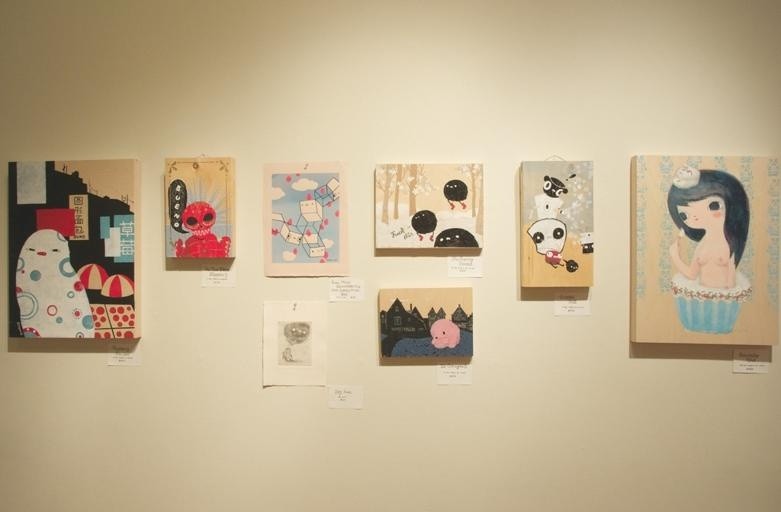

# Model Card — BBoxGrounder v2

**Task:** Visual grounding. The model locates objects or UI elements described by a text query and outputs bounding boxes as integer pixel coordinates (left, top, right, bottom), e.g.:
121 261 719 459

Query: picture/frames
262 161 350 278
